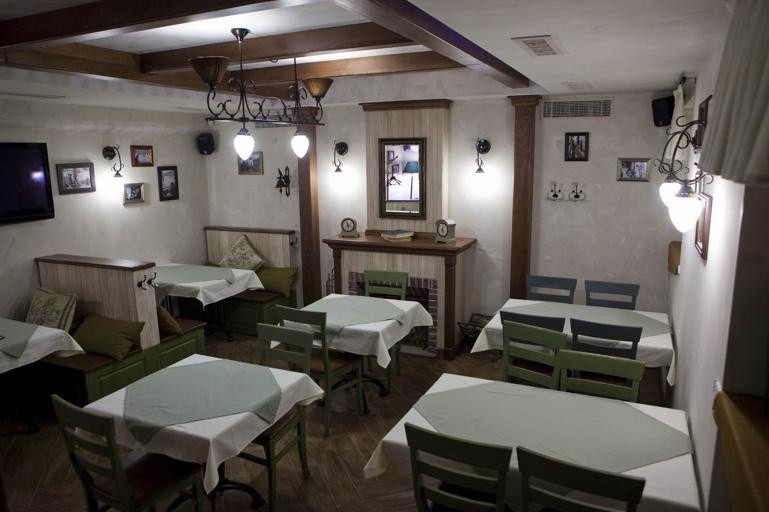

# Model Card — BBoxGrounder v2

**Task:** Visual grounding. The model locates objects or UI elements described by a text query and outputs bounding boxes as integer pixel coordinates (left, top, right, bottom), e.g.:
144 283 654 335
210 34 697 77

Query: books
381 230 414 243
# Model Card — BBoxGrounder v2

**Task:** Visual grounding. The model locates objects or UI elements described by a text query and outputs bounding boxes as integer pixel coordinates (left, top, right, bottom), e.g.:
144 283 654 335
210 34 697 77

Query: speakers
197 133 215 155
652 95 675 127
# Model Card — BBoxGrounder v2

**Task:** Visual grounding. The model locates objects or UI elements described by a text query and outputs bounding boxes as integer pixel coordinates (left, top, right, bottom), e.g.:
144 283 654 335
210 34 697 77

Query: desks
0 317 85 432
363 372 700 511
156 262 263 317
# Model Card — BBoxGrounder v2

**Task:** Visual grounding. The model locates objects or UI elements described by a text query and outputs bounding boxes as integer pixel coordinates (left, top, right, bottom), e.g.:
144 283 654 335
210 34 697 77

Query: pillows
155 282 175 304
216 234 266 271
157 303 183 336
71 314 143 361
257 264 299 298
27 286 77 333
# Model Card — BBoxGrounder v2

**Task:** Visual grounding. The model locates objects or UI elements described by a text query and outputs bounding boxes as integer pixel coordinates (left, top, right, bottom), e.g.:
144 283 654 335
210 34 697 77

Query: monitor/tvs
0 142 55 226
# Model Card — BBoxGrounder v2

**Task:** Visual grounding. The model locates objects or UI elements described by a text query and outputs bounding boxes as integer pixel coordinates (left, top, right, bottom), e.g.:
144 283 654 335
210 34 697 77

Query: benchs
42 348 143 406
159 316 205 370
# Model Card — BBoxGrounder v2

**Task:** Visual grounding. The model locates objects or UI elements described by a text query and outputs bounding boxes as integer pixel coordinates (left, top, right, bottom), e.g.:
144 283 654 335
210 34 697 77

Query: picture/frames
123 183 146 204
237 152 264 176
695 193 712 260
564 132 588 161
130 144 154 167
157 166 179 201
378 137 426 220
56 163 96 195
616 158 652 182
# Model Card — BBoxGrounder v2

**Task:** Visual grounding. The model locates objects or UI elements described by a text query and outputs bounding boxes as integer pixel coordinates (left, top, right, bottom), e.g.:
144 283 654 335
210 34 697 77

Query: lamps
185 27 334 163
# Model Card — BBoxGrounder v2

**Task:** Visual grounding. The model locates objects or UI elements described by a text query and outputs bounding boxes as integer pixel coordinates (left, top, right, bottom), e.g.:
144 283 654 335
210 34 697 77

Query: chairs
48 394 205 512
405 423 511 512
498 274 647 400
517 446 647 512
